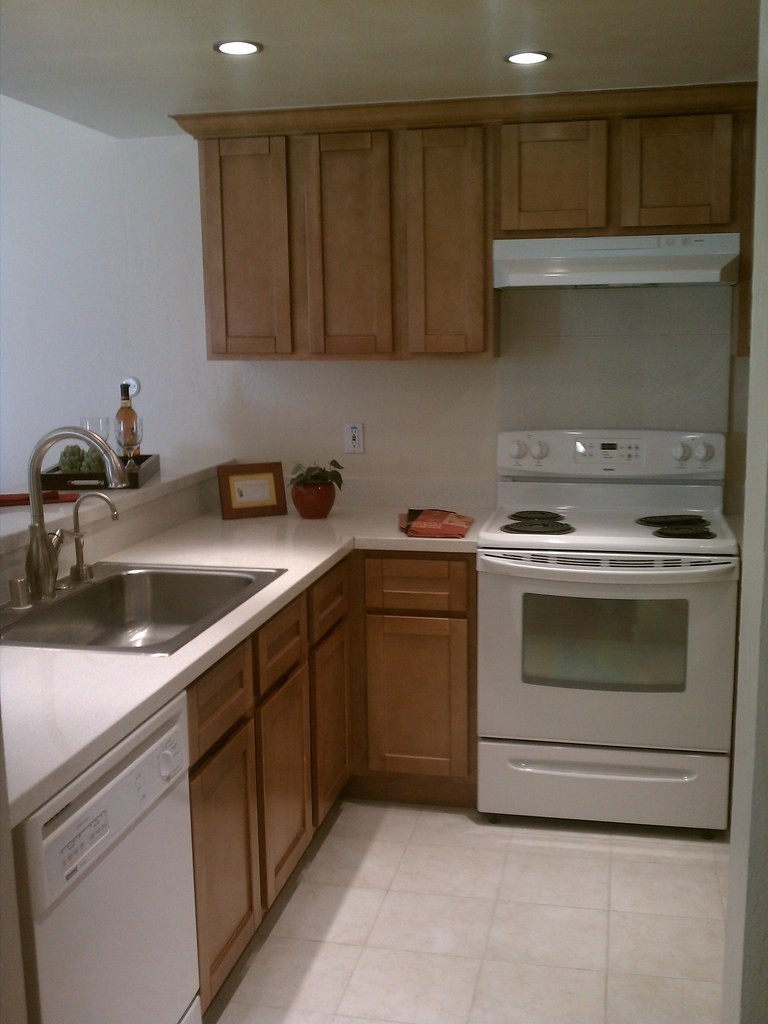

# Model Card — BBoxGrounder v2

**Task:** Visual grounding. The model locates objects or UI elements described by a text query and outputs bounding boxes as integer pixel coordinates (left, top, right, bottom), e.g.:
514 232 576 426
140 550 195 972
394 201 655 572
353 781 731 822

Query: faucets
68 492 120 586
24 427 131 601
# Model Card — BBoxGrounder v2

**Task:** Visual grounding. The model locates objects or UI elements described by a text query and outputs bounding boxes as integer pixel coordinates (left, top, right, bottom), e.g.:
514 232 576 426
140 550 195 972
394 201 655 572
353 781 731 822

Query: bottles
116 384 140 465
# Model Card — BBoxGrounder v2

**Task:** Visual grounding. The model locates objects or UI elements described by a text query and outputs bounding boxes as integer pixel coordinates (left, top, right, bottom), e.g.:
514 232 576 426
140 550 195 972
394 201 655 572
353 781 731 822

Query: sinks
1 558 288 656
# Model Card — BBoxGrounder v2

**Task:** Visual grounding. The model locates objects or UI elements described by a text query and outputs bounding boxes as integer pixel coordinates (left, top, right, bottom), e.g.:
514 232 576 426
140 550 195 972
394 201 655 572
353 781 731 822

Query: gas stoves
478 428 738 555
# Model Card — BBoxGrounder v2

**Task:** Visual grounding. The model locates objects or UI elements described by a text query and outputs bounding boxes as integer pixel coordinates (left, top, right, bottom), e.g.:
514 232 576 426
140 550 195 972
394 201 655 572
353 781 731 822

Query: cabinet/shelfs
358 477 495 810
167 96 499 361
485 80 758 231
102 507 357 1016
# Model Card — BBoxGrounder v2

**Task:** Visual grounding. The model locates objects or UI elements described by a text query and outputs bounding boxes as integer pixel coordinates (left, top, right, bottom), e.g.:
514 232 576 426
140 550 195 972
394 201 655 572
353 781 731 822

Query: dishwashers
14 691 202 1024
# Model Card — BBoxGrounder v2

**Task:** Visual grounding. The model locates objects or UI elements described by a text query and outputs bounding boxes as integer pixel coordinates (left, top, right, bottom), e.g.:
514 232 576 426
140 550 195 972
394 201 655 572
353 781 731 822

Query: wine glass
113 418 143 469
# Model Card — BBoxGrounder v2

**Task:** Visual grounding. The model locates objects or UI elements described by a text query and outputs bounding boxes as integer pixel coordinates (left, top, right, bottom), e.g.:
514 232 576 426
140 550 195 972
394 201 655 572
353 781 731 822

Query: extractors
492 233 739 292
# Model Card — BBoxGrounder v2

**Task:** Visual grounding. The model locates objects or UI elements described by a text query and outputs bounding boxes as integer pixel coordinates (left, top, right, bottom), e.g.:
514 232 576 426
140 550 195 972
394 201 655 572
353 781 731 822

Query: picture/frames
217 459 288 520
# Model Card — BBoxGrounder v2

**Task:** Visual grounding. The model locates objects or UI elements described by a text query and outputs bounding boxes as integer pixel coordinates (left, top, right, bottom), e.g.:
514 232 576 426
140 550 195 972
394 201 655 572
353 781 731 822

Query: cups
81 417 108 447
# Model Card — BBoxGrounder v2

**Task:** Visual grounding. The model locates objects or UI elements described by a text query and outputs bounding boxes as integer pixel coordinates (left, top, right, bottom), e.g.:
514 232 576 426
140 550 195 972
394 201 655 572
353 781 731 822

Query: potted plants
286 457 345 523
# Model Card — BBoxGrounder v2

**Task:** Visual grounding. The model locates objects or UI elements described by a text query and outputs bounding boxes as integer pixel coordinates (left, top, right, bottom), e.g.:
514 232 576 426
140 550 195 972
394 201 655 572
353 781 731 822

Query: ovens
476 547 740 830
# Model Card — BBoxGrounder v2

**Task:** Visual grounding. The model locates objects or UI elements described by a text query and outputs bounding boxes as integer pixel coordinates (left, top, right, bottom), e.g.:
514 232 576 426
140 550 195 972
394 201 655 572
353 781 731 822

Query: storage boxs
39 454 162 490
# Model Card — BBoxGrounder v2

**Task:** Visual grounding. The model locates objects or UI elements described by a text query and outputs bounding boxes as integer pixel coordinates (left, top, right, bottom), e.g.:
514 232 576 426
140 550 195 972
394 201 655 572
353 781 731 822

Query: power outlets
346 422 365 455
